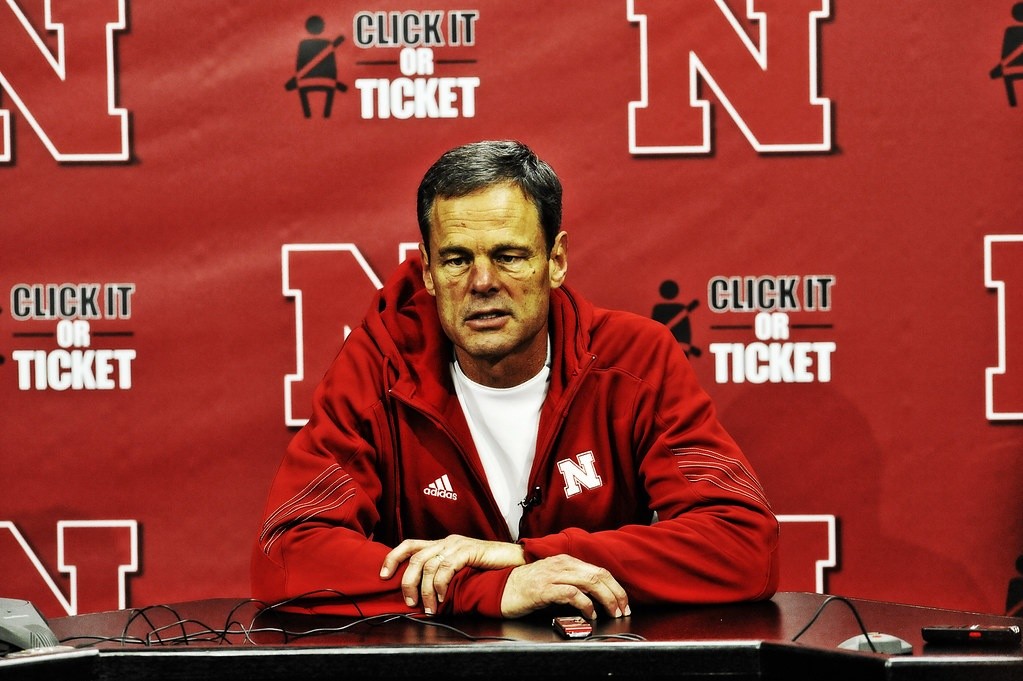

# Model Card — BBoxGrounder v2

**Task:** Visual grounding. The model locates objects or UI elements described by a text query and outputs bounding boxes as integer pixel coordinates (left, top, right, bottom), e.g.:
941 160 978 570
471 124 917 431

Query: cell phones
922 624 1022 646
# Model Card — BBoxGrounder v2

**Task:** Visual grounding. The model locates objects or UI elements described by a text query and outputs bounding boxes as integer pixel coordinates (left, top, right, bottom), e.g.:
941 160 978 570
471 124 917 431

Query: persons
248 137 780 621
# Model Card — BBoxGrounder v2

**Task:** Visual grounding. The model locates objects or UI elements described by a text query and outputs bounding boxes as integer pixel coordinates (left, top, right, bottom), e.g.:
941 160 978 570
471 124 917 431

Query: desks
32 589 1023 681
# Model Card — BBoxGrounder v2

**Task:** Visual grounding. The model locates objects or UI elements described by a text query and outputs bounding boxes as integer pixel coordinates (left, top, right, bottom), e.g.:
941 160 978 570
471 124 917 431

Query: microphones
532 486 541 507
